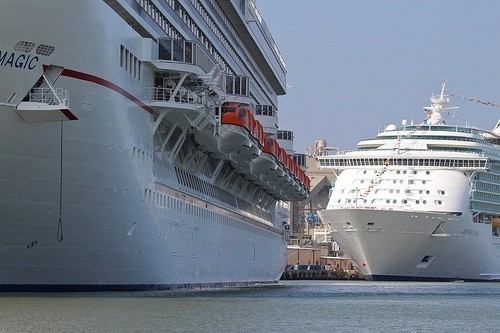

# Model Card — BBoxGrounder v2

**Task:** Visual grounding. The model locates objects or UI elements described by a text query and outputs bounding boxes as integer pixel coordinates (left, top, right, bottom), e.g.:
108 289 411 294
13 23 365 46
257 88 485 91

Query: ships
315 79 499 281
0 0 311 290
